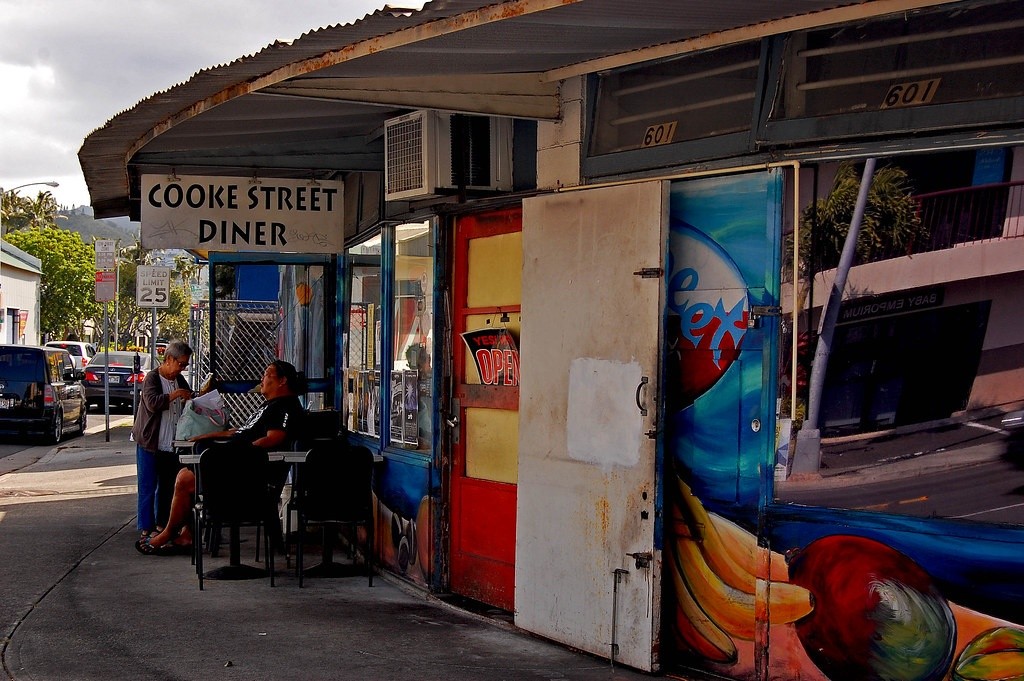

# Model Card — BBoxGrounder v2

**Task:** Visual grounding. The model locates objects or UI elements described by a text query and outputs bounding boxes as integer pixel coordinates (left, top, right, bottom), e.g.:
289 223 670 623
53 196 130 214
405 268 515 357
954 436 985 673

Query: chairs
193 426 375 587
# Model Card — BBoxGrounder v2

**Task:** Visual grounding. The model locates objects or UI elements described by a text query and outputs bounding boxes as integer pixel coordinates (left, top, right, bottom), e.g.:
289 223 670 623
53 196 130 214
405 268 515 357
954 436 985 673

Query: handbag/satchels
174 401 229 440
192 389 226 410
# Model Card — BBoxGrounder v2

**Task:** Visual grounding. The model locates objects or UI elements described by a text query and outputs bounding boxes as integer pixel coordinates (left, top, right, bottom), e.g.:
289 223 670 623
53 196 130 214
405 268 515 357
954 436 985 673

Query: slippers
134 536 193 555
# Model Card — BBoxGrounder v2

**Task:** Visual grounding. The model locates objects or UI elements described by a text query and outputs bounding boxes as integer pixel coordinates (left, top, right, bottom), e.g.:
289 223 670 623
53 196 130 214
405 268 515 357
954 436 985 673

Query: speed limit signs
136 265 171 308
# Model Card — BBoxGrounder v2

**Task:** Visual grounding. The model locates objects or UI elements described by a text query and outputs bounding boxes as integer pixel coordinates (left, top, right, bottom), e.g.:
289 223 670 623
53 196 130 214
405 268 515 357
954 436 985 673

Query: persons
132 340 193 537
135 361 307 554
406 344 432 448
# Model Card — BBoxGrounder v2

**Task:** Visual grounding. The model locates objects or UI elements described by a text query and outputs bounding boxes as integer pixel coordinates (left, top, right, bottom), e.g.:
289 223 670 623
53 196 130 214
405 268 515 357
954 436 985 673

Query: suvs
0 344 88 444
44 341 96 383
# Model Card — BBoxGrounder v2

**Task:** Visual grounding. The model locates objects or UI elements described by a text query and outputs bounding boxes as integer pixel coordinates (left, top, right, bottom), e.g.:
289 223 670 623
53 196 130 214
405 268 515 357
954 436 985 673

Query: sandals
140 524 166 539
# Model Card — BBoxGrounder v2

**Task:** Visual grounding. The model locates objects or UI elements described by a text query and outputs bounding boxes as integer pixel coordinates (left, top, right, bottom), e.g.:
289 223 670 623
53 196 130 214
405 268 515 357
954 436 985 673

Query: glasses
170 354 190 368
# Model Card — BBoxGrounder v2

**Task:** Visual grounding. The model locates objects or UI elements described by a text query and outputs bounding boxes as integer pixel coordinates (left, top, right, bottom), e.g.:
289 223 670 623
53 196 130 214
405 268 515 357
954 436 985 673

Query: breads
200 379 210 395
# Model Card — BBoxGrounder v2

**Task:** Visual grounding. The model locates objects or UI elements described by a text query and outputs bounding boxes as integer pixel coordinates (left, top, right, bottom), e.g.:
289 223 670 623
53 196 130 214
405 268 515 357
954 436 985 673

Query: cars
82 351 162 412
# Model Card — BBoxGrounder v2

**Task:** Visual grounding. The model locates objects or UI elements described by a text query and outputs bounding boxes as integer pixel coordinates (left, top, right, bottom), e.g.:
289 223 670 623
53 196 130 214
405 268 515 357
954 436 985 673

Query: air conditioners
384 110 513 201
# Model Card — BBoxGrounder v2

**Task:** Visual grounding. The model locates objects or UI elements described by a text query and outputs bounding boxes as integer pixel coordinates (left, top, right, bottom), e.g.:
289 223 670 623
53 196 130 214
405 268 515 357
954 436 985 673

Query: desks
172 439 248 544
279 452 384 578
179 452 283 581
